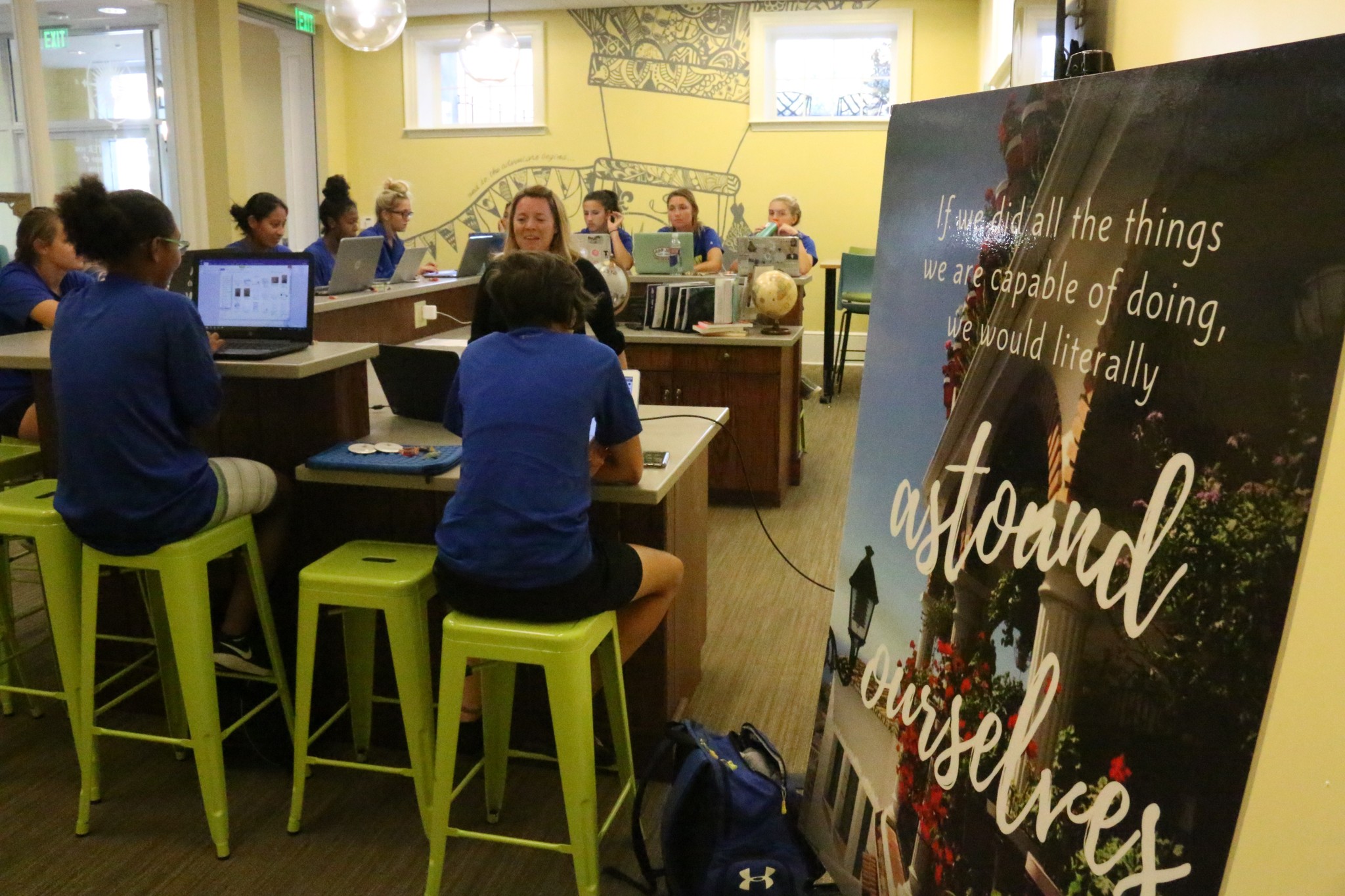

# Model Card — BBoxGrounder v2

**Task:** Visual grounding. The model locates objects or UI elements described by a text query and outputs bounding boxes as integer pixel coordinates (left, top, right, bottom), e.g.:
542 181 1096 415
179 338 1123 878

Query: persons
357 178 438 280
658 188 724 276
0 207 85 442
302 174 359 288
575 189 634 271
730 195 818 298
224 192 292 253
50 177 295 677
467 185 628 370
435 252 683 737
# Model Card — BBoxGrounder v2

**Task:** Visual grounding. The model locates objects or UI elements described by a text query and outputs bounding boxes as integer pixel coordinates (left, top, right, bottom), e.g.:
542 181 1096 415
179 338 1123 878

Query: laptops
371 345 460 423
568 232 611 264
373 247 429 285
469 232 506 274
735 236 802 278
633 233 697 276
187 251 314 360
314 237 385 296
169 247 238 296
424 236 493 278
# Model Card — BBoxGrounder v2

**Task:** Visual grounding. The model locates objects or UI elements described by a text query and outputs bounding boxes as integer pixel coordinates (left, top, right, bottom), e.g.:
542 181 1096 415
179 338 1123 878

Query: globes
751 268 798 335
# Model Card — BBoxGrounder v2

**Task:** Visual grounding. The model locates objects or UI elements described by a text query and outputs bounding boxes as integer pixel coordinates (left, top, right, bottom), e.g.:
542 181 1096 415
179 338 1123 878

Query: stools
0 433 646 896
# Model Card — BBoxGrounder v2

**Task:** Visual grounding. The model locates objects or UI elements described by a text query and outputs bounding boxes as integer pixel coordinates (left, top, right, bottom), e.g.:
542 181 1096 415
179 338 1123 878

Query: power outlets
414 301 427 329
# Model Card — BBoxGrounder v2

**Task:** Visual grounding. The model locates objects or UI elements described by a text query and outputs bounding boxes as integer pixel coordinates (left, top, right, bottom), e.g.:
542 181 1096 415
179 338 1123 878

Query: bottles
669 233 681 276
756 220 778 237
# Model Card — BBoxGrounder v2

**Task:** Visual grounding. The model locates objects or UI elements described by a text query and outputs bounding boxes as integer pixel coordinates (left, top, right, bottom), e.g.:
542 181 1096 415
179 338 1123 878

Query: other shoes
594 735 616 766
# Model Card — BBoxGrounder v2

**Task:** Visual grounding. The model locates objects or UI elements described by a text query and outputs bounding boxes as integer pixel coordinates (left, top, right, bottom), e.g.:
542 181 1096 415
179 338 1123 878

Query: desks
820 259 841 396
0 271 812 779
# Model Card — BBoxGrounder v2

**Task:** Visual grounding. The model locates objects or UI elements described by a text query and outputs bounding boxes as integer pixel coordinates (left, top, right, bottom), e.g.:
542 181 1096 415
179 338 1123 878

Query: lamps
458 0 519 82
325 0 407 51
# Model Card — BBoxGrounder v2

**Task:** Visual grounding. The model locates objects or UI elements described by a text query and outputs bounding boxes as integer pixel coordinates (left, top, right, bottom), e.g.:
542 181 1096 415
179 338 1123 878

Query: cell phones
641 451 668 468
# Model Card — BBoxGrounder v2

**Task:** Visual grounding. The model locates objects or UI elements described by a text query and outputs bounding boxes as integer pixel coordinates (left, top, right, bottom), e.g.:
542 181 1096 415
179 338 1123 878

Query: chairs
832 247 876 394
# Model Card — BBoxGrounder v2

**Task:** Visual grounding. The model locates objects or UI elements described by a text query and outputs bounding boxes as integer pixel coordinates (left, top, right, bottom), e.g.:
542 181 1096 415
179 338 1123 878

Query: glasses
387 209 414 218
162 238 190 255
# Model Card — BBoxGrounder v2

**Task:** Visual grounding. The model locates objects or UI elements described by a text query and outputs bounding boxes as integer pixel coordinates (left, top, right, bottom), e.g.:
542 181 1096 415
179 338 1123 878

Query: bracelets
609 229 618 235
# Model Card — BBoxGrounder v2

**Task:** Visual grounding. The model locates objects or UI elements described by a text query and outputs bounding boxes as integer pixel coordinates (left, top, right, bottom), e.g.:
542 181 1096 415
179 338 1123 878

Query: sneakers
213 629 319 689
